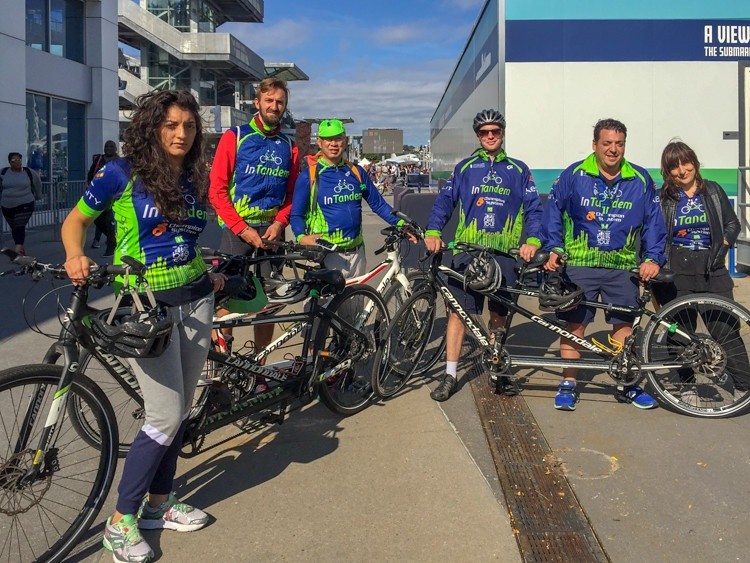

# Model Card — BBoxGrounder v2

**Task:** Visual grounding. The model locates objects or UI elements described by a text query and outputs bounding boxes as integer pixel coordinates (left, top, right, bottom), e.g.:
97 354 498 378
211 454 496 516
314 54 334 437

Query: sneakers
554 380 576 411
92 240 101 248
103 513 154 563
488 374 517 395
137 490 210 532
680 383 702 408
430 371 458 402
733 387 749 402
253 376 269 394
618 384 659 409
102 246 115 257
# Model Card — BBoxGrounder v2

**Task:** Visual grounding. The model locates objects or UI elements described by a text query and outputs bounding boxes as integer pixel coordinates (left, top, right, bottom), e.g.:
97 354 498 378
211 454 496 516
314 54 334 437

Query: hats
317 119 346 138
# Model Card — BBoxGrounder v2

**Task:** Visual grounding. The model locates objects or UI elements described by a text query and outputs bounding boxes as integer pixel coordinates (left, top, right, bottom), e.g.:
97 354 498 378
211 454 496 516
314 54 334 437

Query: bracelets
274 221 282 228
238 225 249 236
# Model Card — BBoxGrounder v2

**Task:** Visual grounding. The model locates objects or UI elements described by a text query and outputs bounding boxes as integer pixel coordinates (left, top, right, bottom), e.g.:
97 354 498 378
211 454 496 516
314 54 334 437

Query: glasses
478 129 503 136
9 158 22 163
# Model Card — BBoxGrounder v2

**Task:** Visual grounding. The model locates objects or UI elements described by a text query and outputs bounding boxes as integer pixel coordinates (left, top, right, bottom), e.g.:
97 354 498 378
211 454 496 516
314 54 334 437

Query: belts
344 242 364 253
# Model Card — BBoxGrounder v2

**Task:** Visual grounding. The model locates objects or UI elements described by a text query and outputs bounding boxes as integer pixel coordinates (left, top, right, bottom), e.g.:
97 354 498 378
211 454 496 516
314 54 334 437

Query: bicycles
378 178 395 195
0 210 750 563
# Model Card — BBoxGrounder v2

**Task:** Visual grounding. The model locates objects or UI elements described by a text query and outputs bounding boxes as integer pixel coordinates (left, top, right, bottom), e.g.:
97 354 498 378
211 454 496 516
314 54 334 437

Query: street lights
420 139 431 174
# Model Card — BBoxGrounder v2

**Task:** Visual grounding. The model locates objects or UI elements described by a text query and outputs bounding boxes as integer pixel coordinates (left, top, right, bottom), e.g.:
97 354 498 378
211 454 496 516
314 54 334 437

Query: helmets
472 109 506 133
218 277 268 314
463 253 502 295
539 281 584 313
91 305 173 358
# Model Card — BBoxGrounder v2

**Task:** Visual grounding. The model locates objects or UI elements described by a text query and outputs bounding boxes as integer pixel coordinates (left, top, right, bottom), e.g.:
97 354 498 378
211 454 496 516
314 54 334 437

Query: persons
206 79 301 396
0 152 42 262
363 160 430 193
94 206 117 258
646 137 750 407
288 120 419 359
62 89 229 563
423 107 544 400
88 140 120 248
542 117 667 410
